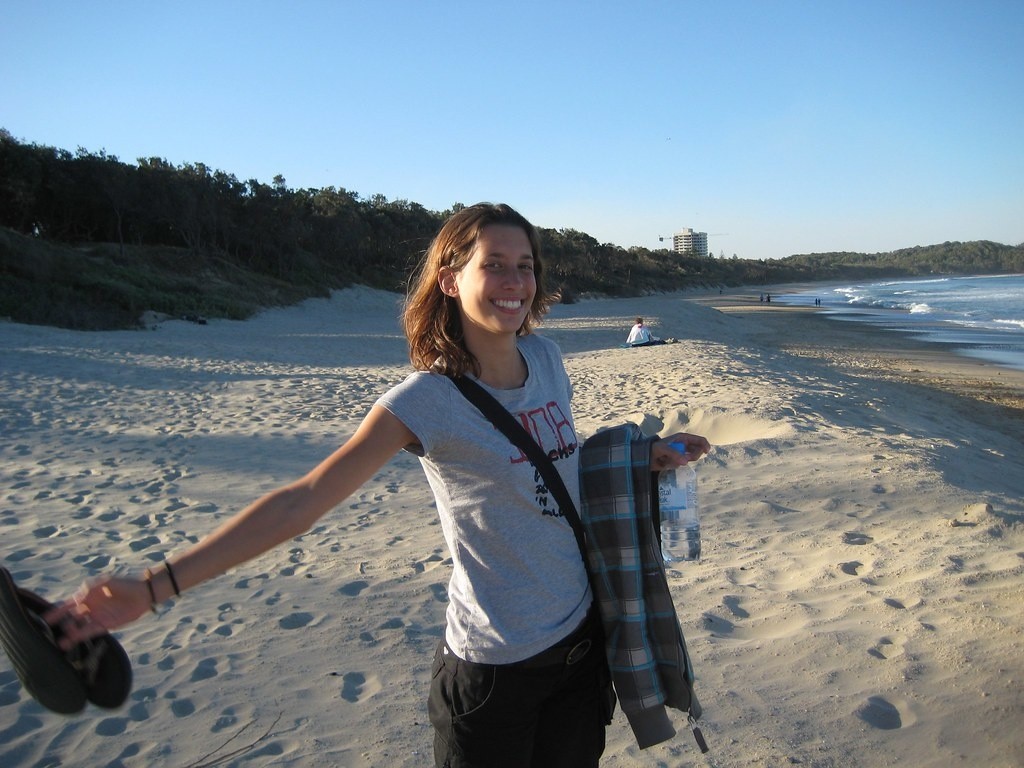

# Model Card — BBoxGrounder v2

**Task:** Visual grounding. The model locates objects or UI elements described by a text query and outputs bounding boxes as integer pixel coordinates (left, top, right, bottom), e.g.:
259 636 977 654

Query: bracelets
162 558 180 595
143 567 158 614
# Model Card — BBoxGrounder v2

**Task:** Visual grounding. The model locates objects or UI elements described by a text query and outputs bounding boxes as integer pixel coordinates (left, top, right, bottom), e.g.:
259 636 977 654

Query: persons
719 289 821 306
45 202 711 768
626 318 666 347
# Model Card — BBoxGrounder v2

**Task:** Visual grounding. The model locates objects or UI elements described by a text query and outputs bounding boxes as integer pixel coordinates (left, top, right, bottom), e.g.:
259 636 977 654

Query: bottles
657 442 702 564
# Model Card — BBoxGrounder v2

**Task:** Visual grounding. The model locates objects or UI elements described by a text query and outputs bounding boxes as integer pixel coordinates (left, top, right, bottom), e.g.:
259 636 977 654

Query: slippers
0 567 133 716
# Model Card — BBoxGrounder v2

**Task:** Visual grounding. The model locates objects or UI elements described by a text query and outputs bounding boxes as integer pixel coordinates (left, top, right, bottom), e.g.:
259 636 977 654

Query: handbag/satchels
606 680 617 725
